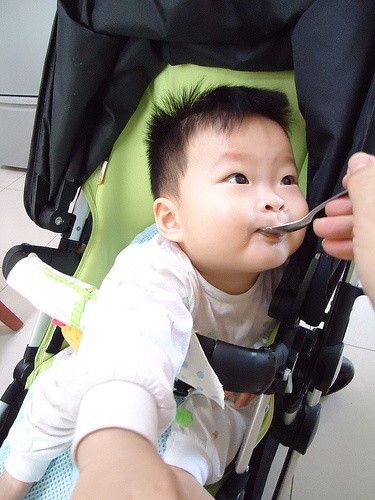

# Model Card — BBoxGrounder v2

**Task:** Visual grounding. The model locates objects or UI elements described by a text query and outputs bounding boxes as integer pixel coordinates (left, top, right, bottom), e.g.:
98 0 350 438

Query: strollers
1 0 375 500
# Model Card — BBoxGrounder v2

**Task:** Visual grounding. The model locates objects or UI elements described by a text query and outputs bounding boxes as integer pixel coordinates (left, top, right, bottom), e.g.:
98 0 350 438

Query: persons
311 151 375 308
0 81 308 500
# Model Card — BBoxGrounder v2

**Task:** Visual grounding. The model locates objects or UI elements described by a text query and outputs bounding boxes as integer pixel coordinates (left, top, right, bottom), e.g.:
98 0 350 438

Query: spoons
260 188 350 233
